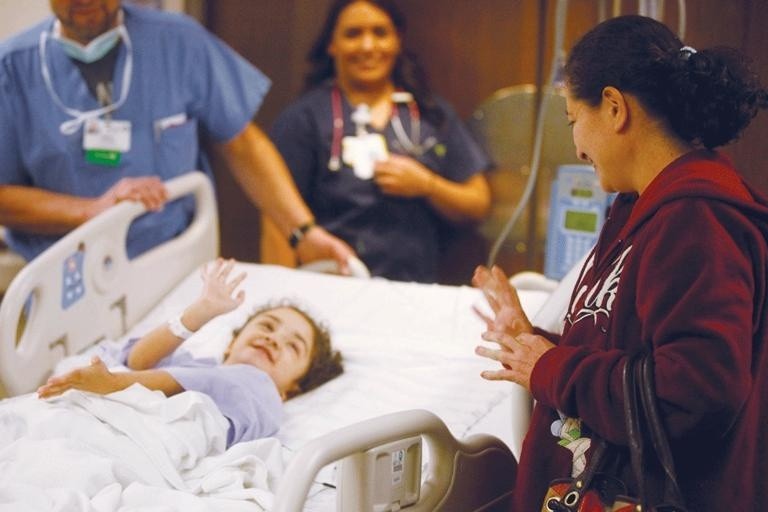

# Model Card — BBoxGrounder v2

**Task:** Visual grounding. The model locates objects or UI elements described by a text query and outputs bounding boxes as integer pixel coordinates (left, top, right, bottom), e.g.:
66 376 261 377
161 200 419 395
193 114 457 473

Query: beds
0 169 597 511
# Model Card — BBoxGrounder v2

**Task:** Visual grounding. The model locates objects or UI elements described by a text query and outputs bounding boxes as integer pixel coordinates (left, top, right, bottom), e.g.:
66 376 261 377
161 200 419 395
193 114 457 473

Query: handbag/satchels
536 347 692 512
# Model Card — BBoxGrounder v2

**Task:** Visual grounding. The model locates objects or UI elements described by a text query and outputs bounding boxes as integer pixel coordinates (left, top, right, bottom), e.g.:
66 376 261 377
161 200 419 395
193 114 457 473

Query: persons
256 3 498 284
30 250 349 458
0 1 372 278
459 13 768 510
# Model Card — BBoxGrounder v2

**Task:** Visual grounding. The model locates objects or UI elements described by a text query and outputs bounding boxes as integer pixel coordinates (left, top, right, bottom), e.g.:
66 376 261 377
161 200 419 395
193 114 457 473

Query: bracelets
167 307 198 341
285 219 325 250
